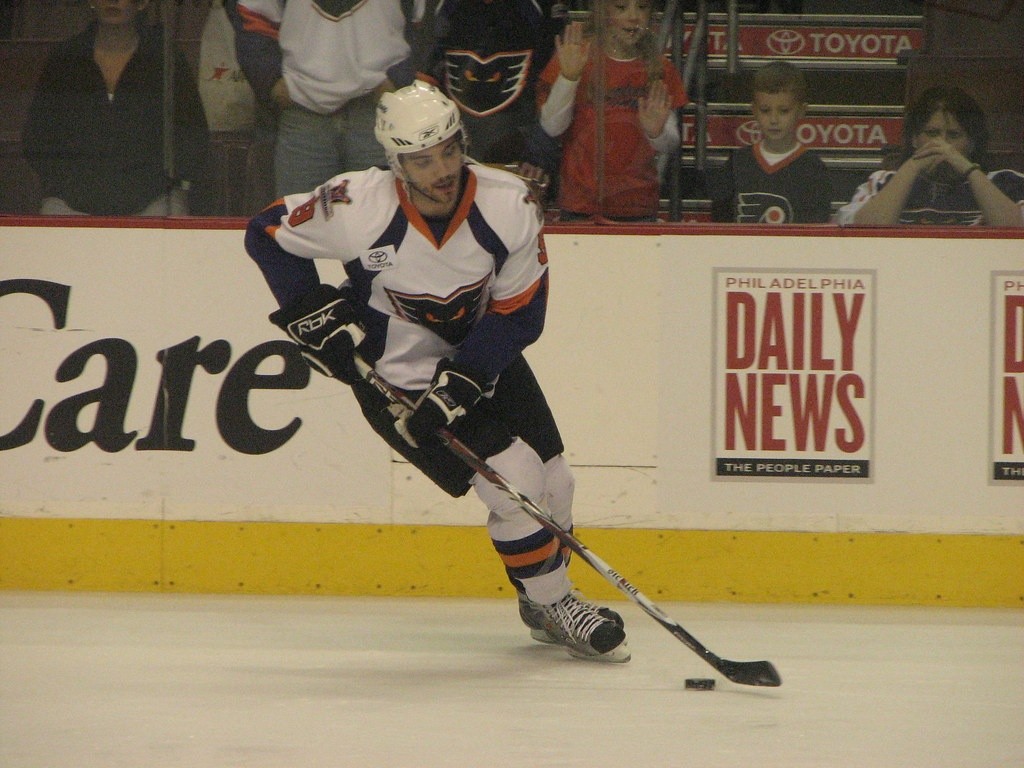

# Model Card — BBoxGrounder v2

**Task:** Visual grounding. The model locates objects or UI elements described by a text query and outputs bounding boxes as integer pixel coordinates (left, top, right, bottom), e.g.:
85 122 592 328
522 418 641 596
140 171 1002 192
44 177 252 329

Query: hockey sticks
353 353 784 688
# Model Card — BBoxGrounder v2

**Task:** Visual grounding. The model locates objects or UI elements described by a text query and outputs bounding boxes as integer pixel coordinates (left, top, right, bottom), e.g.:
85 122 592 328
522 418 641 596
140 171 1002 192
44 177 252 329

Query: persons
532 0 688 225
711 60 835 223
19 0 214 218
223 0 416 200
241 78 638 665
832 85 1024 227
415 0 548 164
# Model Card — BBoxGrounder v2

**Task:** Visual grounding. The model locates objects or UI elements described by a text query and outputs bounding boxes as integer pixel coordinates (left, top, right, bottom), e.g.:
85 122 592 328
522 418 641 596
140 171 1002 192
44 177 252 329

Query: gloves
268 284 382 385
377 356 500 455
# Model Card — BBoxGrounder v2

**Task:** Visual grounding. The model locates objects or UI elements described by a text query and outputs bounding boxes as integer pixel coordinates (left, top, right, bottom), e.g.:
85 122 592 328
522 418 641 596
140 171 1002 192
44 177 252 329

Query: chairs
0 0 250 215
896 51 1024 174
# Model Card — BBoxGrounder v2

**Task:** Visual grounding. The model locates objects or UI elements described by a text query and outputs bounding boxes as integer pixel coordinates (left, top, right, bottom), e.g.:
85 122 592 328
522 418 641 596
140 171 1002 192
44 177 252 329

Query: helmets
373 79 466 182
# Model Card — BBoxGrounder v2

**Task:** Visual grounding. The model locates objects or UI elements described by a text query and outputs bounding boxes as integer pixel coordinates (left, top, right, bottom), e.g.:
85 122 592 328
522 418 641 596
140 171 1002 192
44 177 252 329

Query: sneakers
530 549 625 643
504 569 631 662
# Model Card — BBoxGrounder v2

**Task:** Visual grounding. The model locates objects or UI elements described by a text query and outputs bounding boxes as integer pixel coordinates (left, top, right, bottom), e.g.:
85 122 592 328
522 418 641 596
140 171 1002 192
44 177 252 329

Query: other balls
684 678 715 691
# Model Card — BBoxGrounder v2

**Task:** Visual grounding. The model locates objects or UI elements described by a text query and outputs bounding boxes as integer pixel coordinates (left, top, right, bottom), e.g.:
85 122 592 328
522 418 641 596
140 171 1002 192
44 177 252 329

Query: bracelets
960 162 981 183
